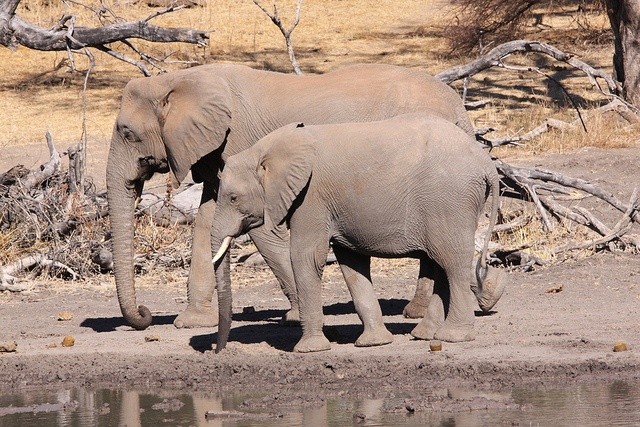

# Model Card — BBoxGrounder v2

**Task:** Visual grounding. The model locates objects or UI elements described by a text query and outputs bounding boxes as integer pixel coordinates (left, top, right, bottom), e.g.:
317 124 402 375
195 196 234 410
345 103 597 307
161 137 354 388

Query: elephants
210 111 499 352
104 64 509 331
